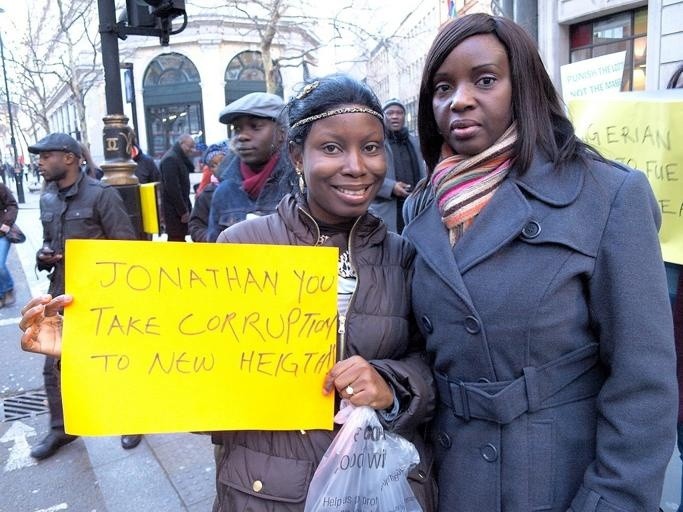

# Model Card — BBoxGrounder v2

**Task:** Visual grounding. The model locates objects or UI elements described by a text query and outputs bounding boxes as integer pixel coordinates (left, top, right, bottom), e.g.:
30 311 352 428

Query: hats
219 93 287 124
28 134 81 157
380 98 405 112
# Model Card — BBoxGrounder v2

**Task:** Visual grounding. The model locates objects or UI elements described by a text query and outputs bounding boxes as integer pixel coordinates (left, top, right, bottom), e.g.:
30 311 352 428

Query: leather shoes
121 435 141 448
30 429 77 457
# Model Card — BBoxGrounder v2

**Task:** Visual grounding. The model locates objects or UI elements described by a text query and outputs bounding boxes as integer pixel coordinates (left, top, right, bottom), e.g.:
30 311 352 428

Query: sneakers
0 290 15 309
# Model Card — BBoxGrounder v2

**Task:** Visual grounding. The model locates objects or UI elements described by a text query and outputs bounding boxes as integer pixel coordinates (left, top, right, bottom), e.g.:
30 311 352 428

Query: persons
0 163 40 309
398 14 683 511
16 76 434 512
25 87 300 459
662 64 681 470
360 96 429 235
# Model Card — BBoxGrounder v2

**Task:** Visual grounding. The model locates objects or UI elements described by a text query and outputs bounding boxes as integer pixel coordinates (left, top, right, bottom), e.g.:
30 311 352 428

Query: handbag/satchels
6 223 26 243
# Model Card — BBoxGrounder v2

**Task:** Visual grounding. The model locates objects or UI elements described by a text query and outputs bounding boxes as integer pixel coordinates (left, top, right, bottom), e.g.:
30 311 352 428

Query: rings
345 385 355 395
